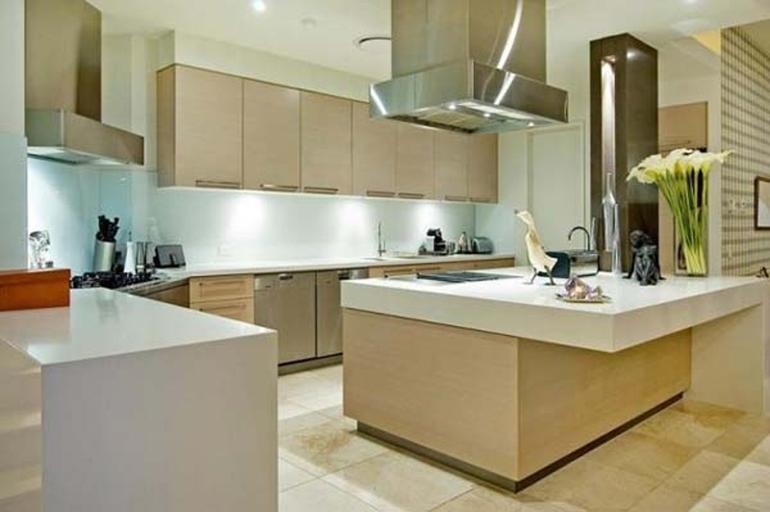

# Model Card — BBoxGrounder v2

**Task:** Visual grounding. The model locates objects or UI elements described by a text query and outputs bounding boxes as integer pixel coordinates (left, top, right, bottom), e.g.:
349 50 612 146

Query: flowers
625 146 737 277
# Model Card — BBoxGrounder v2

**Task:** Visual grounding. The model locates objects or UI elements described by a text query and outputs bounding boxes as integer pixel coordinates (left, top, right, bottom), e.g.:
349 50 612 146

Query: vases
673 207 710 278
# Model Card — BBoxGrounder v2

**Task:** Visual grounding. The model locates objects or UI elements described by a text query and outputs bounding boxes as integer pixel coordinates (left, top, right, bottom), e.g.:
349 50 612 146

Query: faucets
568 226 591 251
377 222 387 255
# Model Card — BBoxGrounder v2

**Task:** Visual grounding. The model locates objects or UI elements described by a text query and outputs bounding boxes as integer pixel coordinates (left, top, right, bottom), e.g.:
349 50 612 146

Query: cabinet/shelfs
156 63 301 194
16 268 341 507
299 85 398 199
398 121 501 205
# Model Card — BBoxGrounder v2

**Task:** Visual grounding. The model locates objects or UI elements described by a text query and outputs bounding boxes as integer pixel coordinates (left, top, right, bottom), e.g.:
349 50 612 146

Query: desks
341 267 767 493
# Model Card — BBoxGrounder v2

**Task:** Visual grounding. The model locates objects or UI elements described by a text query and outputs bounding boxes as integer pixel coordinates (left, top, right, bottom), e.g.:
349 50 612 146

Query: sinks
560 249 600 262
363 255 431 261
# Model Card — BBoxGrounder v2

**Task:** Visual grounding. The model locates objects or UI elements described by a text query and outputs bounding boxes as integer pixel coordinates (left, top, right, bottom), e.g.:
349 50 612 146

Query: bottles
136 240 155 265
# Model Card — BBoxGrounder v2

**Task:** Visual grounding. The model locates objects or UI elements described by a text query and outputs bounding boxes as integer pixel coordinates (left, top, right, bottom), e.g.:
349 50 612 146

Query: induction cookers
380 267 521 288
70 271 162 293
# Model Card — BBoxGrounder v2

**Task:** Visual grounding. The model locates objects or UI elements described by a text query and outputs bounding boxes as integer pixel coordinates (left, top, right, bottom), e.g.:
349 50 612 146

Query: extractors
368 0 570 139
24 2 147 170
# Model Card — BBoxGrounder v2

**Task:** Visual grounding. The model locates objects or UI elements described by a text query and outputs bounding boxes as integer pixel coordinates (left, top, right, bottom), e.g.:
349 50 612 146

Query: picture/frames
754 175 770 230
